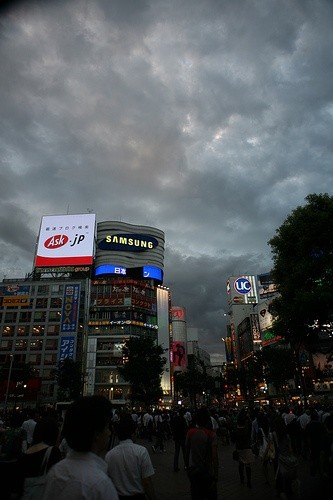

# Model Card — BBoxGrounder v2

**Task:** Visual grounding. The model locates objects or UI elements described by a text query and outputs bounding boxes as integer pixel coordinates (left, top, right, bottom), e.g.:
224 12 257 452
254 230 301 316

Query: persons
170 343 188 374
1 378 332 499
172 310 184 320
